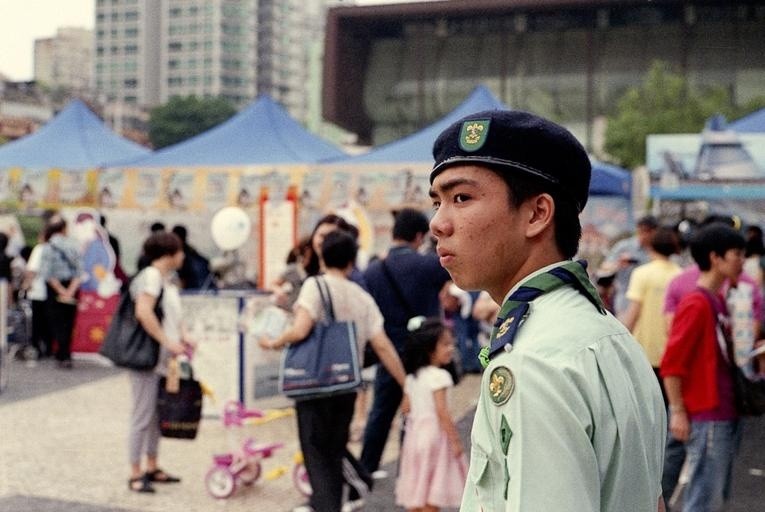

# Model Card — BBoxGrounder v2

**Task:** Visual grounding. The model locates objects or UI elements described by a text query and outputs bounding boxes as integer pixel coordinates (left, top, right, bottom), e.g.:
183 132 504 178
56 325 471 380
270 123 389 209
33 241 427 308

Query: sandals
145 469 181 484
128 477 156 493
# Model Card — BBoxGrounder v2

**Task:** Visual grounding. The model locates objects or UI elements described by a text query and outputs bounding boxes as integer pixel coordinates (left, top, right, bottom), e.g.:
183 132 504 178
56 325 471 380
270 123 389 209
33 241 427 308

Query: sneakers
372 469 391 480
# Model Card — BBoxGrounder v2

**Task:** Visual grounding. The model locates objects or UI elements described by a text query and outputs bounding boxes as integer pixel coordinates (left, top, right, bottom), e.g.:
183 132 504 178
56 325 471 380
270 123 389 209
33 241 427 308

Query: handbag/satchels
278 275 362 402
156 361 202 440
97 286 165 371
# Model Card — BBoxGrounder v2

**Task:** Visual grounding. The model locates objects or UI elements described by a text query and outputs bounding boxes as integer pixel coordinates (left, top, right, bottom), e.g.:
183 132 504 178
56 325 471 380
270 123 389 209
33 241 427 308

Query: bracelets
669 403 685 412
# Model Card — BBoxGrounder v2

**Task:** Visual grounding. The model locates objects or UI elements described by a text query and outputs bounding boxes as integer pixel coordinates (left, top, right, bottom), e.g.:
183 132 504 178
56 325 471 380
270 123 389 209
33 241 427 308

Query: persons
394 322 475 512
130 232 197 492
305 215 353 316
171 226 208 291
258 231 410 512
42 224 85 369
0 233 14 392
619 232 687 511
743 224 765 291
428 110 667 512
657 223 746 512
20 227 48 361
636 215 696 268
274 236 311 308
663 215 765 512
592 219 654 314
346 206 464 510
15 248 36 344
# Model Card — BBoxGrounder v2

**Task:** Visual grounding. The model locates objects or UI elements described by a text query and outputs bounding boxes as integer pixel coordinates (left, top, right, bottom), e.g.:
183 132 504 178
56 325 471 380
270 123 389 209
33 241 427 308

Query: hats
429 111 592 213
